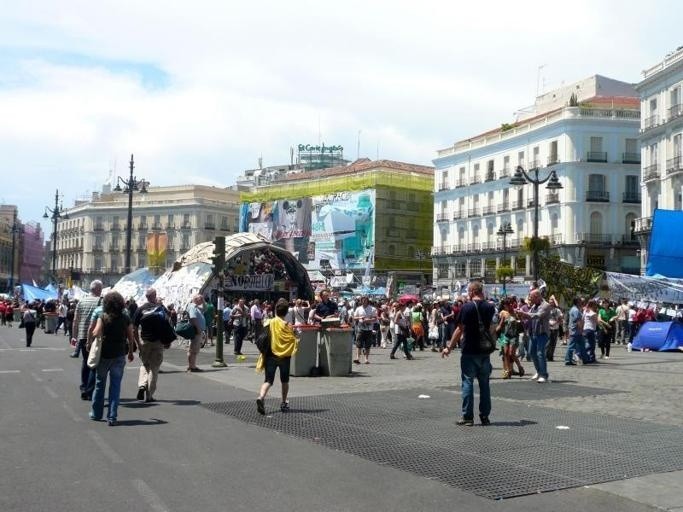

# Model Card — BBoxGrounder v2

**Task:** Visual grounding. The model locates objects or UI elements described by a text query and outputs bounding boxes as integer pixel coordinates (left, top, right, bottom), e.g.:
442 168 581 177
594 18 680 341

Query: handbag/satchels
176 310 197 339
254 324 271 358
86 334 107 369
472 299 495 356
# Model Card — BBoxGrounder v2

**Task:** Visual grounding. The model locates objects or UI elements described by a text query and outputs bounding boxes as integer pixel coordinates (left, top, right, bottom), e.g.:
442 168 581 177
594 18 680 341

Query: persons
249 298 274 340
20 298 58 342
167 301 183 328
353 296 378 365
284 290 361 326
377 300 466 360
490 289 561 383
202 295 219 347
61 279 138 425
256 297 302 416
441 281 496 427
615 298 638 345
565 292 615 366
132 288 172 404
0 289 22 325
185 294 207 372
223 296 252 356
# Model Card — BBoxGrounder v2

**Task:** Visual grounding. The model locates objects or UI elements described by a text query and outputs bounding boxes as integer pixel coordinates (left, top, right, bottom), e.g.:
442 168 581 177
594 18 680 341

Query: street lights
496 219 514 290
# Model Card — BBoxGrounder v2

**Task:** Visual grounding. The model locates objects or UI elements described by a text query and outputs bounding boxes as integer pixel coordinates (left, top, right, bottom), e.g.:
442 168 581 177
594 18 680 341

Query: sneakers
456 419 474 427
537 377 546 382
136 386 144 401
280 402 292 411
531 374 538 379
256 396 265 415
481 416 490 425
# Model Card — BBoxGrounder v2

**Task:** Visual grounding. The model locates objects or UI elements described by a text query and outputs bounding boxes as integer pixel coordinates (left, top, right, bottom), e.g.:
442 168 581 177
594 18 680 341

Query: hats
433 300 439 304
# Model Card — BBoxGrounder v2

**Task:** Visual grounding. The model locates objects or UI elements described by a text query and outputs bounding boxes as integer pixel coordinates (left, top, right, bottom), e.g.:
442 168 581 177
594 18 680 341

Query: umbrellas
398 294 419 306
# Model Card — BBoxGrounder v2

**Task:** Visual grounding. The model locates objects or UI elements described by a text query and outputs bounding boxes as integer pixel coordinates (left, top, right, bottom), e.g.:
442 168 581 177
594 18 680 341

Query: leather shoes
565 360 575 365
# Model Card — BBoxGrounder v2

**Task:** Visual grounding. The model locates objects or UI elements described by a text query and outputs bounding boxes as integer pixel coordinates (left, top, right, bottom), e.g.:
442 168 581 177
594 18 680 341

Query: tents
630 319 682 351
22 283 59 303
